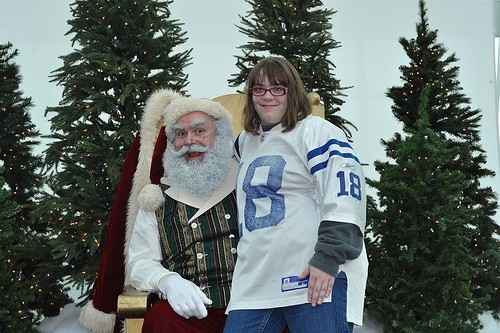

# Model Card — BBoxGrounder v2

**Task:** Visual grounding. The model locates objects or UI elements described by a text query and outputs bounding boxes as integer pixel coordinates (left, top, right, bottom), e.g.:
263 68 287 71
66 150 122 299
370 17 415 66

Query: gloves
162 274 213 320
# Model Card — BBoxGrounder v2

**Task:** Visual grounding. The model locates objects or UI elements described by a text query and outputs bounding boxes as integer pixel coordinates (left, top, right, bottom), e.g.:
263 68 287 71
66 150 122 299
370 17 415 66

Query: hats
163 98 231 143
126 88 232 232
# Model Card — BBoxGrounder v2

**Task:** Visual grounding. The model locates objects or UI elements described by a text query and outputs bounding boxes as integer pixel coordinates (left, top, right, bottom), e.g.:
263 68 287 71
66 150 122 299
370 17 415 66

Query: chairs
117 91 324 333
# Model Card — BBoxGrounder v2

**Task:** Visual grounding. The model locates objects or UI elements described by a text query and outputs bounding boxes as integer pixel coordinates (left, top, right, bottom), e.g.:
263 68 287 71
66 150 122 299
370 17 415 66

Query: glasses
171 133 218 148
249 87 289 96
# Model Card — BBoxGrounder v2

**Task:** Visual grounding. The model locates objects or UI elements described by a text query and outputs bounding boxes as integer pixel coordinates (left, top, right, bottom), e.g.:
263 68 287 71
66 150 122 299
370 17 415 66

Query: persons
127 97 241 333
222 56 370 333
